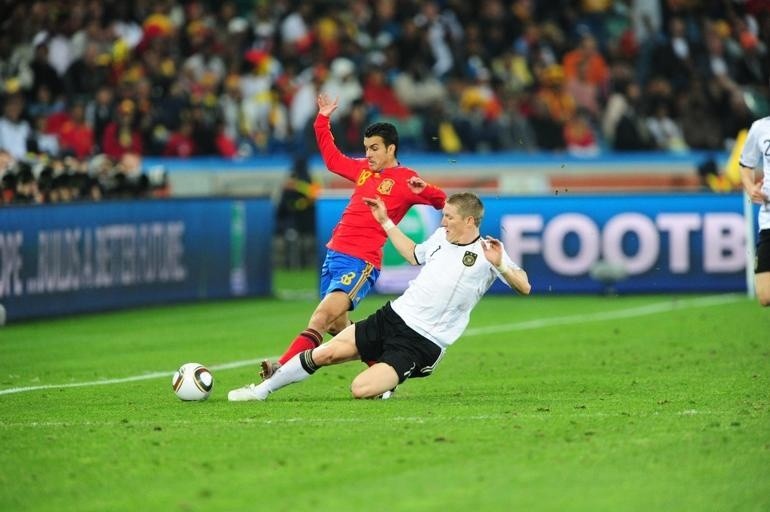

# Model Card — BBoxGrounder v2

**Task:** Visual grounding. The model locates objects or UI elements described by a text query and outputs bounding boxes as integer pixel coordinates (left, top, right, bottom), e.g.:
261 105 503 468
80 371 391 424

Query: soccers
171 362 213 403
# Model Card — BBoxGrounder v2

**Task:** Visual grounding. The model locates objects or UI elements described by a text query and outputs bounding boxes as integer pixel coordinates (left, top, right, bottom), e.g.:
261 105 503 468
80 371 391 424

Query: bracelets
381 217 395 232
496 262 508 275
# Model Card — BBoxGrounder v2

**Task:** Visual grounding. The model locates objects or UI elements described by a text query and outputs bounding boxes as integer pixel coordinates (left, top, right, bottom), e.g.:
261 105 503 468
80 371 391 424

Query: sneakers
259 359 278 381
228 385 268 401
380 386 400 399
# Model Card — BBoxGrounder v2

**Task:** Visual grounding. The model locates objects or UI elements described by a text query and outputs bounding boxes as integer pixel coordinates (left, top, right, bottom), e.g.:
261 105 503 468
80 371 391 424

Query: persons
229 93 447 381
227 193 531 402
0 0 770 206
740 116 770 307
